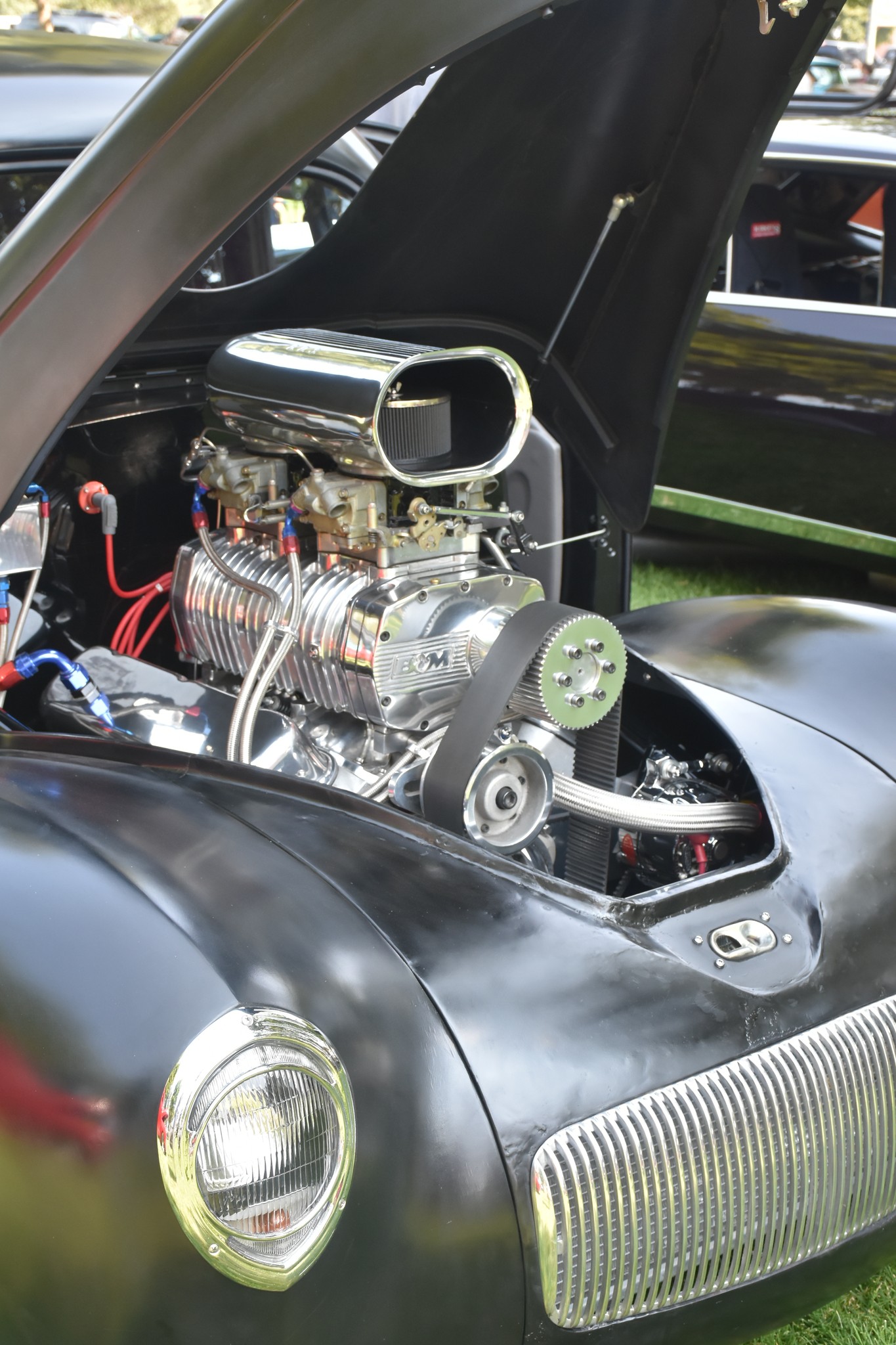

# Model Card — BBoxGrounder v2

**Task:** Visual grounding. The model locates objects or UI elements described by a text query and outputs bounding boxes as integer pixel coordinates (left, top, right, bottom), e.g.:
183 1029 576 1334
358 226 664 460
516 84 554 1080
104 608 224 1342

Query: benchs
725 182 879 308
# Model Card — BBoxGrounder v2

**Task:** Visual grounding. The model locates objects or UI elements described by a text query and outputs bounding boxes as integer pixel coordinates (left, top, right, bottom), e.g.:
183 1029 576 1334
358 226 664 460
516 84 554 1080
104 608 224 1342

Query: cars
0 0 896 1345
